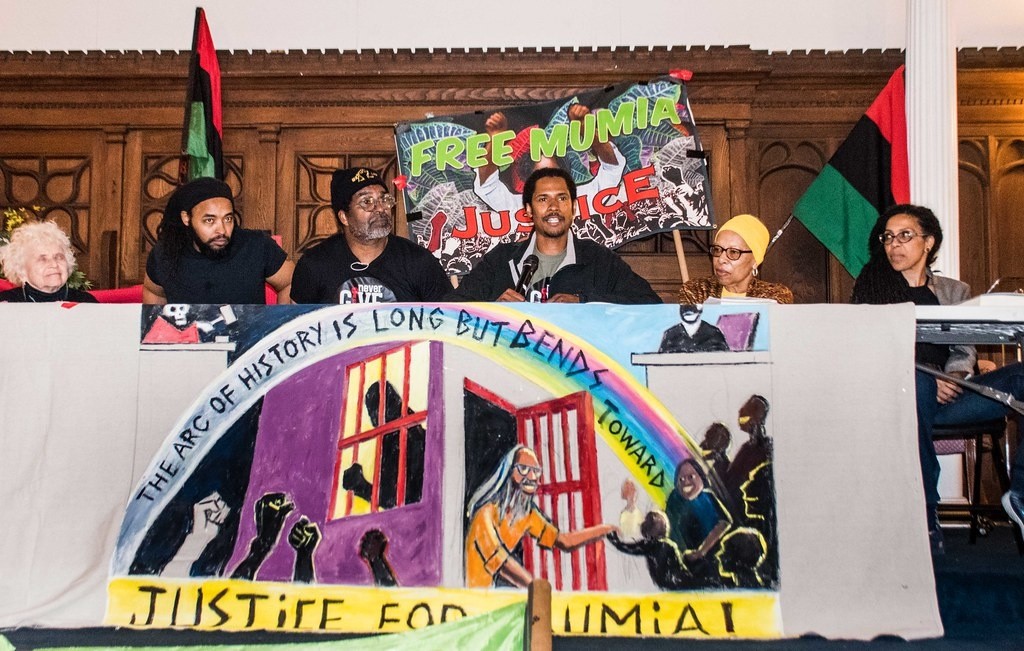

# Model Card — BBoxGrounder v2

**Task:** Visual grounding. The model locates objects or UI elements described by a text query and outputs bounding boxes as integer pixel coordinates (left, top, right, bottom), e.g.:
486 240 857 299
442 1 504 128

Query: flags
179 7 223 182
791 62 910 280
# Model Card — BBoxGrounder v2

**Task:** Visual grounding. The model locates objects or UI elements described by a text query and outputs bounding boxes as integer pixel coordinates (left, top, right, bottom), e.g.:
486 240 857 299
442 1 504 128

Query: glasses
344 194 396 212
878 230 929 245
709 244 753 261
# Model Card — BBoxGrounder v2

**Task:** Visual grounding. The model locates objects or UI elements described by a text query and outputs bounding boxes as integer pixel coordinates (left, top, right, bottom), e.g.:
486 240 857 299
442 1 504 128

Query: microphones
516 255 539 297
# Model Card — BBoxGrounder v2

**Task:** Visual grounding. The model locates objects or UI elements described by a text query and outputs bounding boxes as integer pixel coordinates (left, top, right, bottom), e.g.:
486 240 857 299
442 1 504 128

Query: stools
929 418 1024 557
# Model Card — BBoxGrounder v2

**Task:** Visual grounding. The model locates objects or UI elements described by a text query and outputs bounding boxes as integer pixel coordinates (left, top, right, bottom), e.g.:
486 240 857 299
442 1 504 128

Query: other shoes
928 507 943 550
1001 492 1024 538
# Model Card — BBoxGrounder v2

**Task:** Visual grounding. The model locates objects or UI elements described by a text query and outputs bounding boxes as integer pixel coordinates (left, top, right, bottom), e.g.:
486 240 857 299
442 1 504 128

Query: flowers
0 205 95 292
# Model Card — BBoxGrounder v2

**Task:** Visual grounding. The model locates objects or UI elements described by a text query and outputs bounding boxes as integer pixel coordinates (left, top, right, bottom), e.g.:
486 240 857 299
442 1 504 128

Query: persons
290 167 456 304
142 176 296 305
850 204 1024 545
676 213 794 303
445 167 666 305
472 104 628 233
0 218 99 302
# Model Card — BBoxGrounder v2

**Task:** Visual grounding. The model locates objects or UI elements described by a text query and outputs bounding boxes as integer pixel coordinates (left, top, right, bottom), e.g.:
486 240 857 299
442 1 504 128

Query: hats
177 176 235 219
714 215 770 266
330 167 389 227
